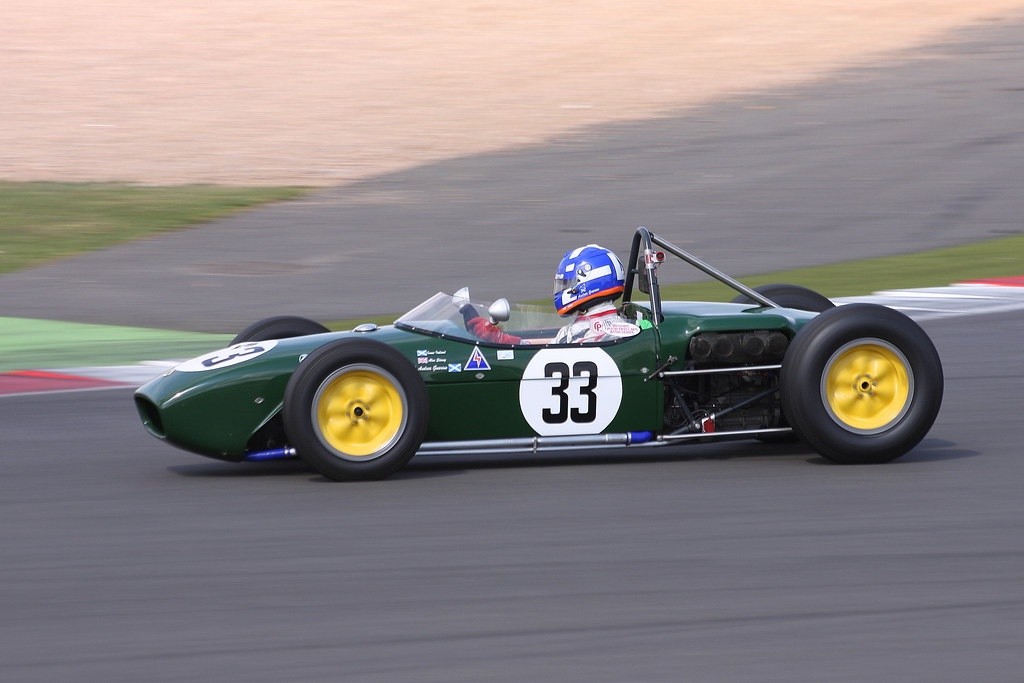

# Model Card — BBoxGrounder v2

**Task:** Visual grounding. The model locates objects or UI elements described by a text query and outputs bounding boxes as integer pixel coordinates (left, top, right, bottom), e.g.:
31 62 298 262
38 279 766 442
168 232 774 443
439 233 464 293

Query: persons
467 244 643 345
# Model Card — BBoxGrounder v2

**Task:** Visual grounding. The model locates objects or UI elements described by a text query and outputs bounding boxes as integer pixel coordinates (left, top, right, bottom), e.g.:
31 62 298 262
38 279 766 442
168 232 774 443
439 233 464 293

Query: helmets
552 244 626 317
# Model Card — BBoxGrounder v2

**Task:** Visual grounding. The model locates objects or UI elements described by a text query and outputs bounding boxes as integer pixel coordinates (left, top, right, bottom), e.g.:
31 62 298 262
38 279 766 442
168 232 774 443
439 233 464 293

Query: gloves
467 316 521 345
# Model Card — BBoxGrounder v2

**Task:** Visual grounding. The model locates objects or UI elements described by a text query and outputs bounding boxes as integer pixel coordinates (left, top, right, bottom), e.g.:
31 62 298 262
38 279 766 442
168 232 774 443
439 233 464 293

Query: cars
135 227 949 484
